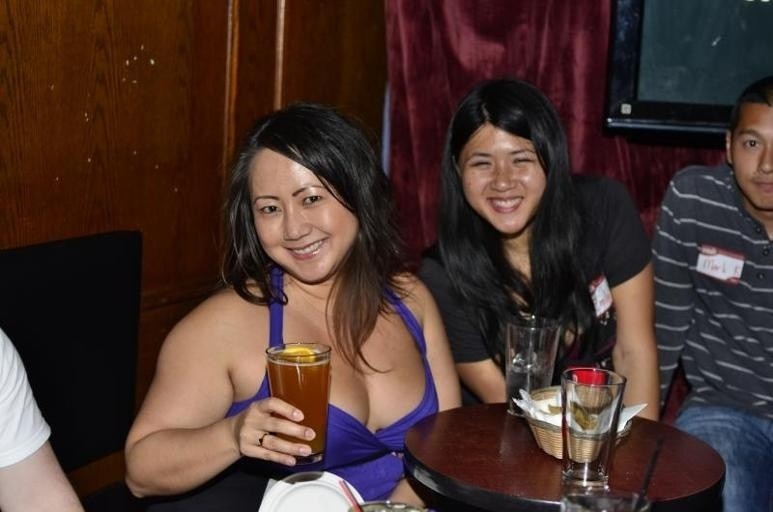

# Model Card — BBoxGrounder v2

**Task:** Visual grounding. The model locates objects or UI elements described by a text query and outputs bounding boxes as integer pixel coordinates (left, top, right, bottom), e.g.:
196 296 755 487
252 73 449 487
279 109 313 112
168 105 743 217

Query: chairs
1 227 148 511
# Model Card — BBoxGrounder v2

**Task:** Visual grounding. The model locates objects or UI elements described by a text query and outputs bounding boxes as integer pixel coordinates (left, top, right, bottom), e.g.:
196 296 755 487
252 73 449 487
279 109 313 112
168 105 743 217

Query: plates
256 470 365 512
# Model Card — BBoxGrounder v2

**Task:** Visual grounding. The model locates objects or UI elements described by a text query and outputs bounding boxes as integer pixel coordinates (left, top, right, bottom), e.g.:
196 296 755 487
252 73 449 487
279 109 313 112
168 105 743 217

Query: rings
259 433 267 448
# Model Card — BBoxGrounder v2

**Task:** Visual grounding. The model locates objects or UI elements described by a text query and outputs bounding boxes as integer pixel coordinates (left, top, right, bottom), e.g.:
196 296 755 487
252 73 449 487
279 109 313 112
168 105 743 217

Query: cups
501 313 565 419
559 365 631 494
263 339 333 467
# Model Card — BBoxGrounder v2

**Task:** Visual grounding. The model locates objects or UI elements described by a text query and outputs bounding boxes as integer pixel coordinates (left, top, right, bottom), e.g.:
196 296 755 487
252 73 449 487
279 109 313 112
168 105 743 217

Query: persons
415 76 660 423
0 325 88 511
648 73 773 511
123 101 463 506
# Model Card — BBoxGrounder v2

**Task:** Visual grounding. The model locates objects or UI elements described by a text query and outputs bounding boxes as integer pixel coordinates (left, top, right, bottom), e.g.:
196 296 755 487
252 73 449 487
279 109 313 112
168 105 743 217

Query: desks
402 401 728 512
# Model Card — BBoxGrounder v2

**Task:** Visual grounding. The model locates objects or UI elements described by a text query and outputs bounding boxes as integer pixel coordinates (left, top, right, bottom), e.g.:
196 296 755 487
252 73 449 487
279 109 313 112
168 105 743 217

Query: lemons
282 346 316 363
